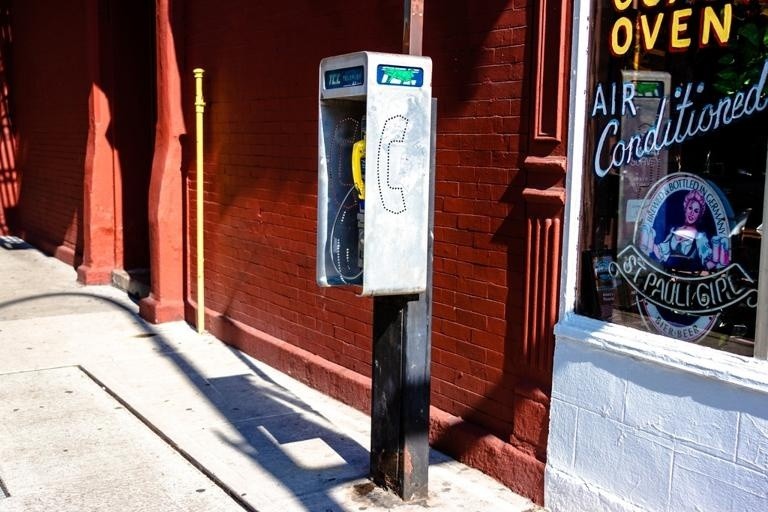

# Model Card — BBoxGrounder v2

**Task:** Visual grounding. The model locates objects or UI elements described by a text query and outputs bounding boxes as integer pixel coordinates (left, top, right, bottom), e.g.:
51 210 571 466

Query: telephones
351 139 366 200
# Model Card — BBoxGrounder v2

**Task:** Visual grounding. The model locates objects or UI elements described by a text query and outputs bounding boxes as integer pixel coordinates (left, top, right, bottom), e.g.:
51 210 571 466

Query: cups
640 223 656 253
710 234 729 267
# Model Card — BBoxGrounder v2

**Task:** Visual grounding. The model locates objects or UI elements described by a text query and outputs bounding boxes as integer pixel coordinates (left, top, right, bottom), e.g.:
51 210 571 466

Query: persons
645 190 724 272
